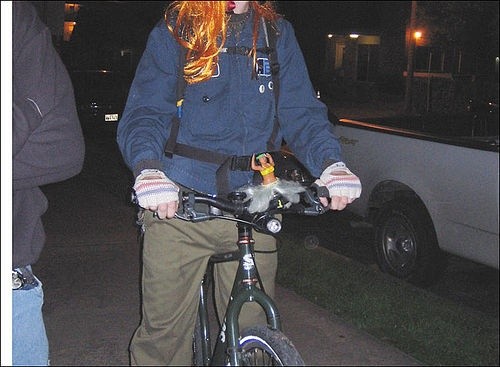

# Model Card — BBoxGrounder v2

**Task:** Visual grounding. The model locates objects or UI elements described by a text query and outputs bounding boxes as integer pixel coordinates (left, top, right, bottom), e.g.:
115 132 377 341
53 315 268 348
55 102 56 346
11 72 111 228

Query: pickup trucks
333 100 499 279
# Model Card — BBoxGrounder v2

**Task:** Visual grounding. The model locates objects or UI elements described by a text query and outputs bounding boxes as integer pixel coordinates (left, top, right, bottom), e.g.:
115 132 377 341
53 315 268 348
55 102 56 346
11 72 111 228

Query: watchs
12 270 28 290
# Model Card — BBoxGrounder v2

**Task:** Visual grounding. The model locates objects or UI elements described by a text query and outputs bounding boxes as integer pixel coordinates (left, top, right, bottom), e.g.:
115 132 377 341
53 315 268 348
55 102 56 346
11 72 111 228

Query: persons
13 0 86 367
116 0 362 367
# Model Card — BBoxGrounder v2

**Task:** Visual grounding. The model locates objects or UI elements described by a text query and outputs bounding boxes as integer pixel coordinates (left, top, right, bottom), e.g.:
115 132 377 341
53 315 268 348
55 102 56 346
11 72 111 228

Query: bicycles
130 179 333 366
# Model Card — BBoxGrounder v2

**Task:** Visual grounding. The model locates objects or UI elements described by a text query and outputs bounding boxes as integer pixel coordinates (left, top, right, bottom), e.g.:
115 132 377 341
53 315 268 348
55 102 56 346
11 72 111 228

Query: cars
66 67 130 131
